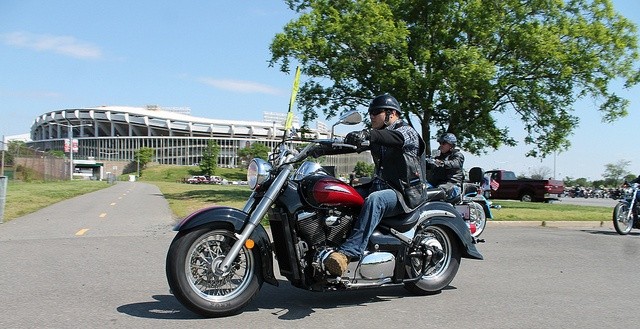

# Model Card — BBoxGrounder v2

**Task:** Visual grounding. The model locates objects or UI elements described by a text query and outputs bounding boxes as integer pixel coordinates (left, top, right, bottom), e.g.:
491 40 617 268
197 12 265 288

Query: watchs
166 110 486 315
425 166 501 239
613 180 640 234
566 184 624 200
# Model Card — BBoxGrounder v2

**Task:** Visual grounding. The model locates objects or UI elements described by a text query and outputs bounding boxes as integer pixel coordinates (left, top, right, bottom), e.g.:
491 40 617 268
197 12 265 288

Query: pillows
437 133 457 146
368 95 402 115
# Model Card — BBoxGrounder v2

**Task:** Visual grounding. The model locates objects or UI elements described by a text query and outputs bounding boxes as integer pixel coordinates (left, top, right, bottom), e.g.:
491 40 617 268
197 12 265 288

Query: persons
426 133 465 202
628 175 640 186
306 95 428 276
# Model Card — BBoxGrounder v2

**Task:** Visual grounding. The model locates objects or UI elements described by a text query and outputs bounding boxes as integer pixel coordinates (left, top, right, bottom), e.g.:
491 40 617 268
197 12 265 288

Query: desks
50 123 93 179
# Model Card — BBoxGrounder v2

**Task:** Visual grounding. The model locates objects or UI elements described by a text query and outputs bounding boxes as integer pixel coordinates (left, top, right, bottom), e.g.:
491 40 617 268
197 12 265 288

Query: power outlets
484 170 565 204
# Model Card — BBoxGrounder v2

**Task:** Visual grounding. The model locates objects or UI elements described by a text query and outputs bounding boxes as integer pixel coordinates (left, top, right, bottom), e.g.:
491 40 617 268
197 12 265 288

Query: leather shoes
345 129 370 149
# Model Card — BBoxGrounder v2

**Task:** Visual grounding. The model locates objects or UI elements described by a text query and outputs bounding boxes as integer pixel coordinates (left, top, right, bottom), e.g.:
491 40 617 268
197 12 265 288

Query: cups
440 142 446 145
370 109 384 116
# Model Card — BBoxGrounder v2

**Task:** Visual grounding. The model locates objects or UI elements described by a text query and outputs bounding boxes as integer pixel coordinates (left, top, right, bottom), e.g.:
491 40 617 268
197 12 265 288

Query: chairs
324 252 349 276
